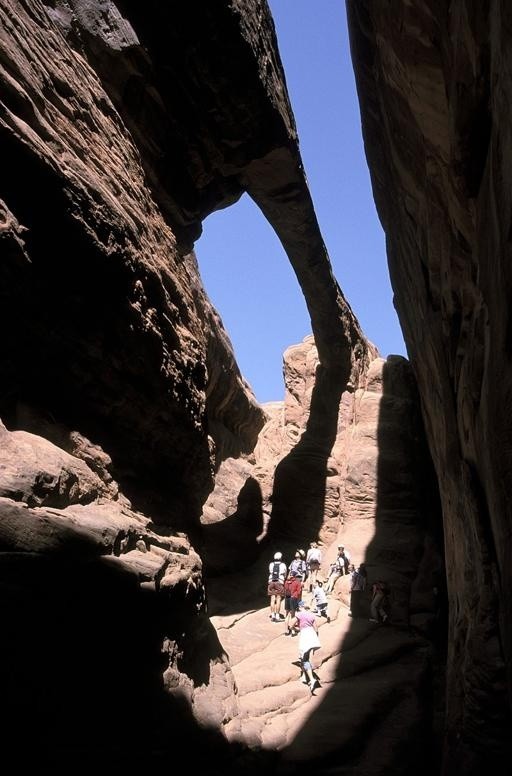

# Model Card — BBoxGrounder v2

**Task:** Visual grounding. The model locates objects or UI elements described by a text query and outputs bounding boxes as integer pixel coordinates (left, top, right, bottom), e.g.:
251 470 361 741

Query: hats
296 548 306 559
274 552 282 560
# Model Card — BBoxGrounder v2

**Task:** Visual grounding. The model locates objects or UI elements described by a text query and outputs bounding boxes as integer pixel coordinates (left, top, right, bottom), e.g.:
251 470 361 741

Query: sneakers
300 677 308 683
271 618 285 622
310 678 317 692
369 618 379 624
383 614 387 622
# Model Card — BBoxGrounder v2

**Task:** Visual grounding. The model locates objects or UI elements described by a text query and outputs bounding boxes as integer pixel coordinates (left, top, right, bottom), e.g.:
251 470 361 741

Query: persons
288 600 320 691
268 542 389 635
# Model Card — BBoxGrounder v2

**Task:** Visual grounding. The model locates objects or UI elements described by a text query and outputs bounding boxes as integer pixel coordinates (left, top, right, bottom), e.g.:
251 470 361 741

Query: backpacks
272 563 280 581
